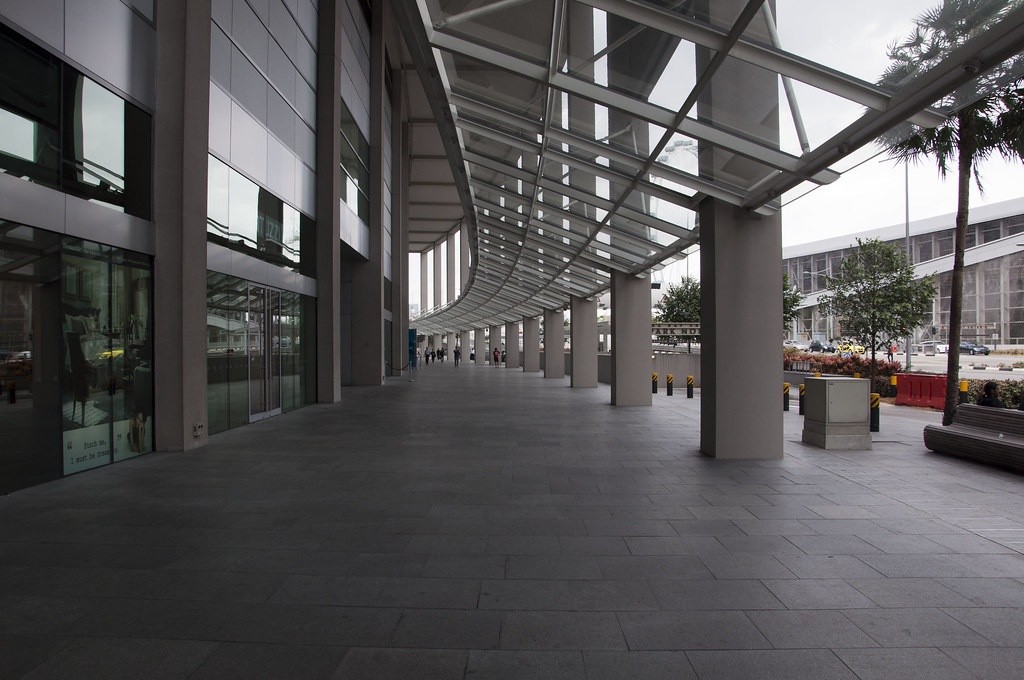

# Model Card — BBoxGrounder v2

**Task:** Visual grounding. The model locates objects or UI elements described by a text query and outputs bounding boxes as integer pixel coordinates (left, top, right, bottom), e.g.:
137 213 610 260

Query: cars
809 340 836 353
959 340 991 356
783 340 809 352
919 341 949 353
837 341 866 355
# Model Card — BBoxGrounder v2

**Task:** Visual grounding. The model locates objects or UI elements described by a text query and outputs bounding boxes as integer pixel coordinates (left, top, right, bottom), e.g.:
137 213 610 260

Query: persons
828 337 833 345
493 347 501 367
424 347 445 364
847 340 853 347
454 345 460 367
886 345 893 362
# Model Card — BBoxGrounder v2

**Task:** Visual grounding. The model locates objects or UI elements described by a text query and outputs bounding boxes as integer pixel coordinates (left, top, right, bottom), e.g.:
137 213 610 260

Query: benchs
924 403 1024 473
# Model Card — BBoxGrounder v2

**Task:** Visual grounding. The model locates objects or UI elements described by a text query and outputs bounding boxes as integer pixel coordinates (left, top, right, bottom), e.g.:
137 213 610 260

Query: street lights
803 266 839 341
